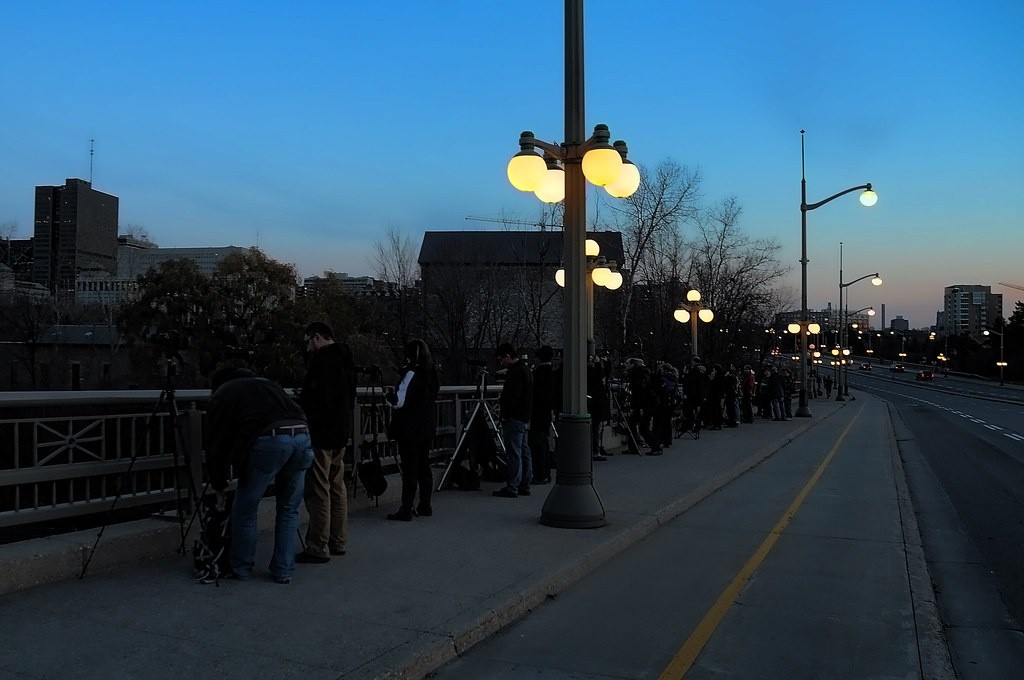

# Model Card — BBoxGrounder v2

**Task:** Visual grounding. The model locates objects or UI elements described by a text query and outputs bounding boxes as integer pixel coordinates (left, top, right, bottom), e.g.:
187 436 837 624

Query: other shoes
593 455 607 461
270 570 289 584
530 476 550 486
772 417 783 422
492 487 518 498
645 447 664 456
517 486 532 496
601 448 614 457
386 509 412 522
662 445 670 448
622 448 633 454
413 505 433 516
785 417 793 421
295 551 330 564
231 569 249 580
329 548 347 556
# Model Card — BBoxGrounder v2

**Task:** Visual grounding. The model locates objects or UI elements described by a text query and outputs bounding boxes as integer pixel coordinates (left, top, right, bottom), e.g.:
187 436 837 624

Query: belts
257 426 310 436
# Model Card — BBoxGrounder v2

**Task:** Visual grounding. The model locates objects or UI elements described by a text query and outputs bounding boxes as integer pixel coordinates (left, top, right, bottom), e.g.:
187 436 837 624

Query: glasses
303 334 316 346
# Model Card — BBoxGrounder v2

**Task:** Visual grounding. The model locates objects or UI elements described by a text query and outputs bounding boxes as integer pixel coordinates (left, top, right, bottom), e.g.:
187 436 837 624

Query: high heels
709 424 722 430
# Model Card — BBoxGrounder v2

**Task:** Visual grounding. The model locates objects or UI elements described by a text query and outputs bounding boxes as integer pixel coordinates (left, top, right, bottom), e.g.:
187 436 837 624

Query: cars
859 363 872 371
916 370 935 381
889 364 904 373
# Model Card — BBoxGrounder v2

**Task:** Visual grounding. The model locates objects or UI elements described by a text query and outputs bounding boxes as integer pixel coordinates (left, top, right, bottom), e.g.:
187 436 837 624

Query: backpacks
191 503 238 584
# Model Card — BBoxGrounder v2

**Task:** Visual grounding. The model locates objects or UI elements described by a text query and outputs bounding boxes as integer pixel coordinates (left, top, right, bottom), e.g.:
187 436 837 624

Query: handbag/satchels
444 457 481 490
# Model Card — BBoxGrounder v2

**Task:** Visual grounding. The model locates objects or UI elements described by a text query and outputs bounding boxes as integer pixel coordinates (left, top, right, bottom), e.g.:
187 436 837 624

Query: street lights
833 304 859 389
674 290 714 363
782 330 799 370
787 129 878 417
813 334 823 375
832 242 883 401
508 0 641 529
890 330 907 362
841 287 876 396
554 239 623 359
930 331 948 377
983 327 1008 386
858 330 881 365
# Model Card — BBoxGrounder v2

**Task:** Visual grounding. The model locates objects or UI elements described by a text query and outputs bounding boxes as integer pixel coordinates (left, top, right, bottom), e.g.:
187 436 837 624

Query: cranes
466 212 564 231
998 282 1024 291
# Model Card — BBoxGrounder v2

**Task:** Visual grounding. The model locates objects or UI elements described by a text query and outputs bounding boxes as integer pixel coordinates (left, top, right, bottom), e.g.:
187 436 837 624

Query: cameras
152 329 190 350
356 364 383 377
466 359 487 367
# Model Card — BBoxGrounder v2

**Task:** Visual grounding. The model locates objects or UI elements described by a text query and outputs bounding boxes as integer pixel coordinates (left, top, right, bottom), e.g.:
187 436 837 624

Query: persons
492 342 534 497
534 343 794 483
298 322 357 564
823 375 833 399
204 365 314 586
381 338 441 520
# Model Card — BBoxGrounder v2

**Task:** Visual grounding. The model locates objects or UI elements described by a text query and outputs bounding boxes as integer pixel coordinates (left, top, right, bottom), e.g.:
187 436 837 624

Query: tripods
601 376 642 457
79 351 203 580
436 371 506 492
345 377 419 517
